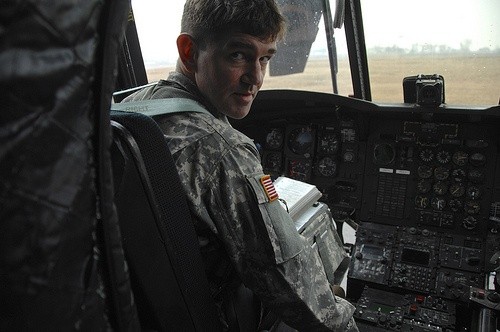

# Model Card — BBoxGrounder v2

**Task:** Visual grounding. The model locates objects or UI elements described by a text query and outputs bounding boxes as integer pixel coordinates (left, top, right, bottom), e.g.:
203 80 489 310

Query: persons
115 0 363 332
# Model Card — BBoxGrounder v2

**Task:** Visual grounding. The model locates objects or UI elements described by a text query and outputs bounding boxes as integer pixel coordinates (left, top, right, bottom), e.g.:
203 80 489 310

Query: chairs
108 109 282 332
